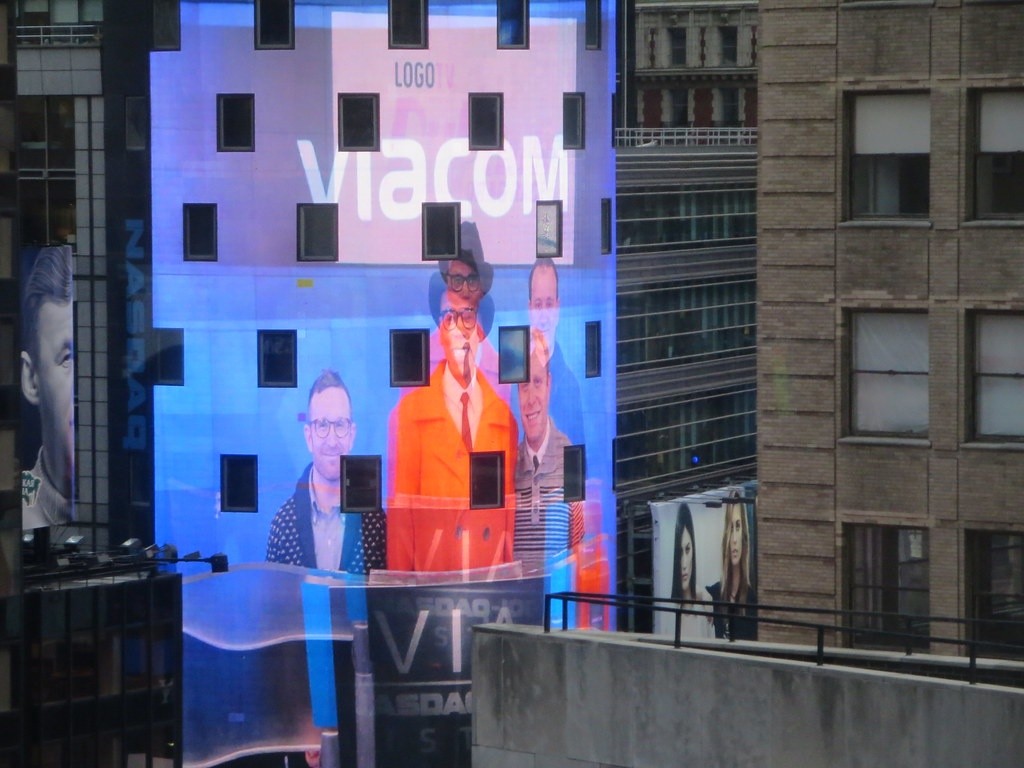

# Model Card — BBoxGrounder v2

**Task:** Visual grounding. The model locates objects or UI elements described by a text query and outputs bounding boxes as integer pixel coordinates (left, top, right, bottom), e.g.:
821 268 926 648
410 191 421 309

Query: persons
433 215 490 324
507 253 583 475
380 270 522 582
11 242 75 534
659 503 717 648
256 366 387 596
491 329 583 594
707 488 757 654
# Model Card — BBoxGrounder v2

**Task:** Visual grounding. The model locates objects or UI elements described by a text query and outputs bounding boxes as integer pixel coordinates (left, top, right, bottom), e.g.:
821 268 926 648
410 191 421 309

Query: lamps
208 552 228 573
